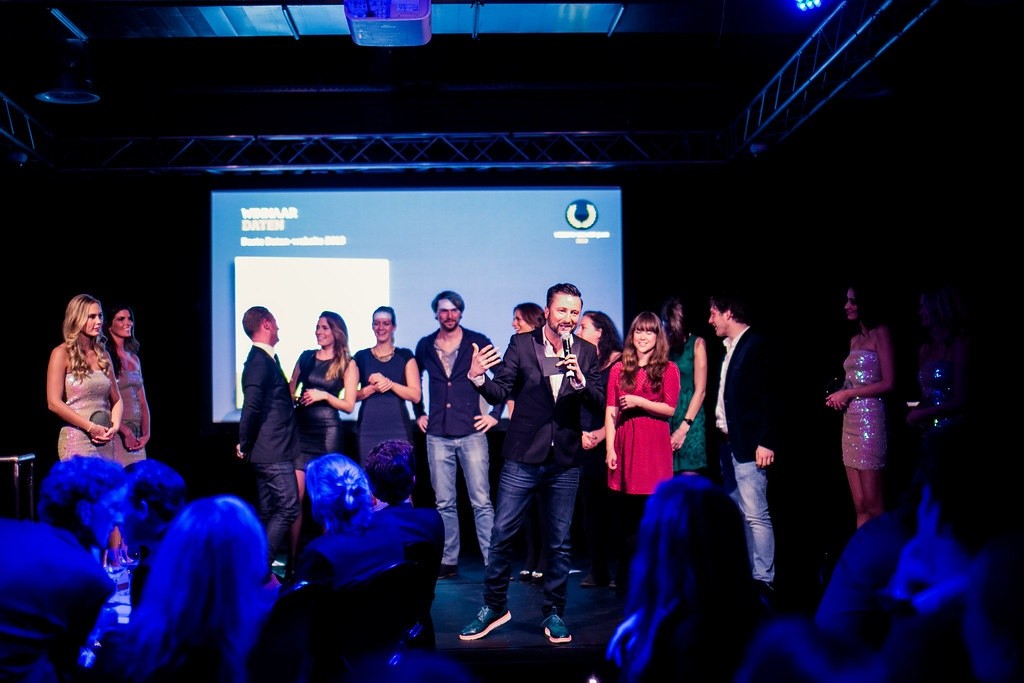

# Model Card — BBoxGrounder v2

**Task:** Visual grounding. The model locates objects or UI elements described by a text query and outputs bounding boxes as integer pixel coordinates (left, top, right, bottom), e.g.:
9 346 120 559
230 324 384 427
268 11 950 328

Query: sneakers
458 604 512 641
540 606 572 643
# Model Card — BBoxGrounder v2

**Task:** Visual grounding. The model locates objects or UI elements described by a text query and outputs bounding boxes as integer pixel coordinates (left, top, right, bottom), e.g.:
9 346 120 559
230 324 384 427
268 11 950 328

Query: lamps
343 0 432 47
33 7 100 105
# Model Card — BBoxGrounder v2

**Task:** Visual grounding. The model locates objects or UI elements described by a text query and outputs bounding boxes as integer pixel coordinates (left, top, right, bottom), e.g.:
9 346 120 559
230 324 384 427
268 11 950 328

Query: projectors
343 0 432 47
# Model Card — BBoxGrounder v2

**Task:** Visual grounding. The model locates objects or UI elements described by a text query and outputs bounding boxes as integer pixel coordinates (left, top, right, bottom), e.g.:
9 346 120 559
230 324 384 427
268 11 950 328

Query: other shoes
608 580 617 591
437 564 458 579
579 574 596 587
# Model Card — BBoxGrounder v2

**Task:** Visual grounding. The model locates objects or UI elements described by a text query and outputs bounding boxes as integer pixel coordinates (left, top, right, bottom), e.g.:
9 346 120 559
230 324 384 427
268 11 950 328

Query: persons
289 311 359 555
706 288 780 590
605 312 679 602
826 279 895 529
506 302 707 591
47 294 124 468
459 284 607 642
0 439 469 683
412 291 505 580
234 306 299 582
907 288 970 495
592 420 1024 683
353 307 421 504
101 301 150 466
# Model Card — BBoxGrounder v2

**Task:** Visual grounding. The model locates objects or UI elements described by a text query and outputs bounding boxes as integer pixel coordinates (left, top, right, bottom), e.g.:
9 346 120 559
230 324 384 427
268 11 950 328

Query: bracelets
87 422 93 432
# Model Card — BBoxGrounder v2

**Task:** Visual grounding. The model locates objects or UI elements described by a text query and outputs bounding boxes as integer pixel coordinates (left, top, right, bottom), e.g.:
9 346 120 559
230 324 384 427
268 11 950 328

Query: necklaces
858 328 876 345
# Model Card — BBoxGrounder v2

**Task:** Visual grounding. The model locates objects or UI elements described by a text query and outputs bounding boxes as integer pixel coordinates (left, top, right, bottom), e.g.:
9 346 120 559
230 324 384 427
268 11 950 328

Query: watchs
683 418 694 425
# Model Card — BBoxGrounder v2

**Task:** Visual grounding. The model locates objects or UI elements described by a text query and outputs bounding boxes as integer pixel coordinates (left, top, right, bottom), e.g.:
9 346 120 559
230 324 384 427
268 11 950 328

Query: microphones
560 331 575 379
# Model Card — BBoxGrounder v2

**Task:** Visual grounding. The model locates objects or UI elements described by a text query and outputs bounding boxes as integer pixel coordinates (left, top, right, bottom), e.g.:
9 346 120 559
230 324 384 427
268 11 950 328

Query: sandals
519 569 545 584
